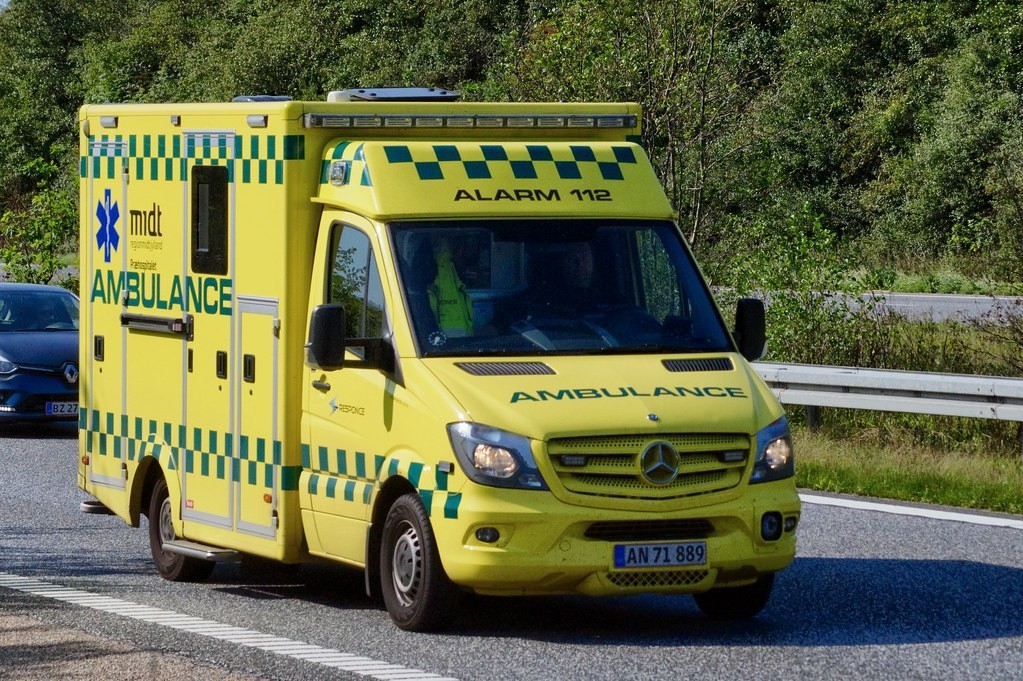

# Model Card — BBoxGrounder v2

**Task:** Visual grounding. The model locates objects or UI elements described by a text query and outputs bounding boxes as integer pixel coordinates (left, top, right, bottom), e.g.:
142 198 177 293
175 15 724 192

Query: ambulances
76 86 801 634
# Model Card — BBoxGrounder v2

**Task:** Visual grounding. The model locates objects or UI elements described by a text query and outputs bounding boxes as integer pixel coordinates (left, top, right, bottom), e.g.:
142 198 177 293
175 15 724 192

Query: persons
502 241 632 336
26 299 60 330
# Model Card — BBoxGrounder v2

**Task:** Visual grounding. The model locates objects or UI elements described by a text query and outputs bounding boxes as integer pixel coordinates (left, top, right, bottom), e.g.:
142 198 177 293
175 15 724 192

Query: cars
0 283 82 421
